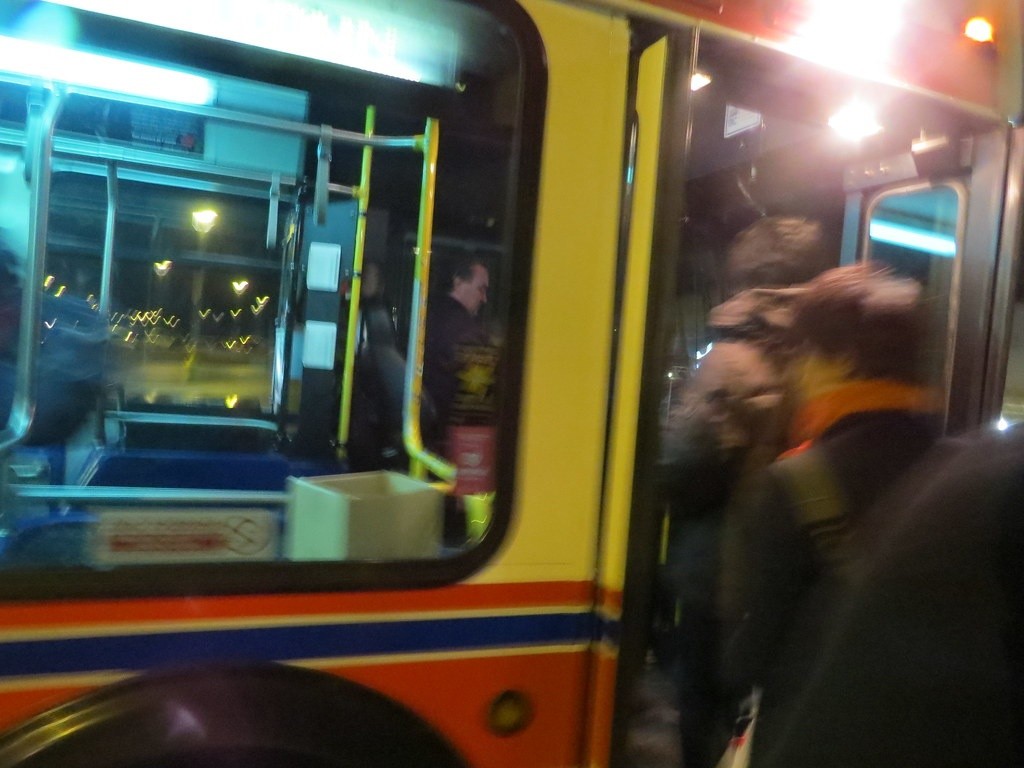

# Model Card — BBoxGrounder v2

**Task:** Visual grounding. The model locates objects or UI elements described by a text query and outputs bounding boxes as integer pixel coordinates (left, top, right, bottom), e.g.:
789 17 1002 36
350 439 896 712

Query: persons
424 260 507 421
356 264 409 372
639 216 1024 768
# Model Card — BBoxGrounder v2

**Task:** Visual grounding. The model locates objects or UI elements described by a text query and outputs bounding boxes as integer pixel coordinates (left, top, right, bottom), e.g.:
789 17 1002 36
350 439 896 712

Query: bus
0 0 1024 768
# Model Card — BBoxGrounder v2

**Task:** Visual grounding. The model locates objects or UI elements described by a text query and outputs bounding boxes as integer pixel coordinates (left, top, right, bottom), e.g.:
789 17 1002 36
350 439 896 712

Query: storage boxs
285 469 445 563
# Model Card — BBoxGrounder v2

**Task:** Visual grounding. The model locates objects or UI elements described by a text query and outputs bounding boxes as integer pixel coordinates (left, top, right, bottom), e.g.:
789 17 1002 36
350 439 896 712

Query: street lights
184 210 219 379
154 260 173 330
233 281 248 339
